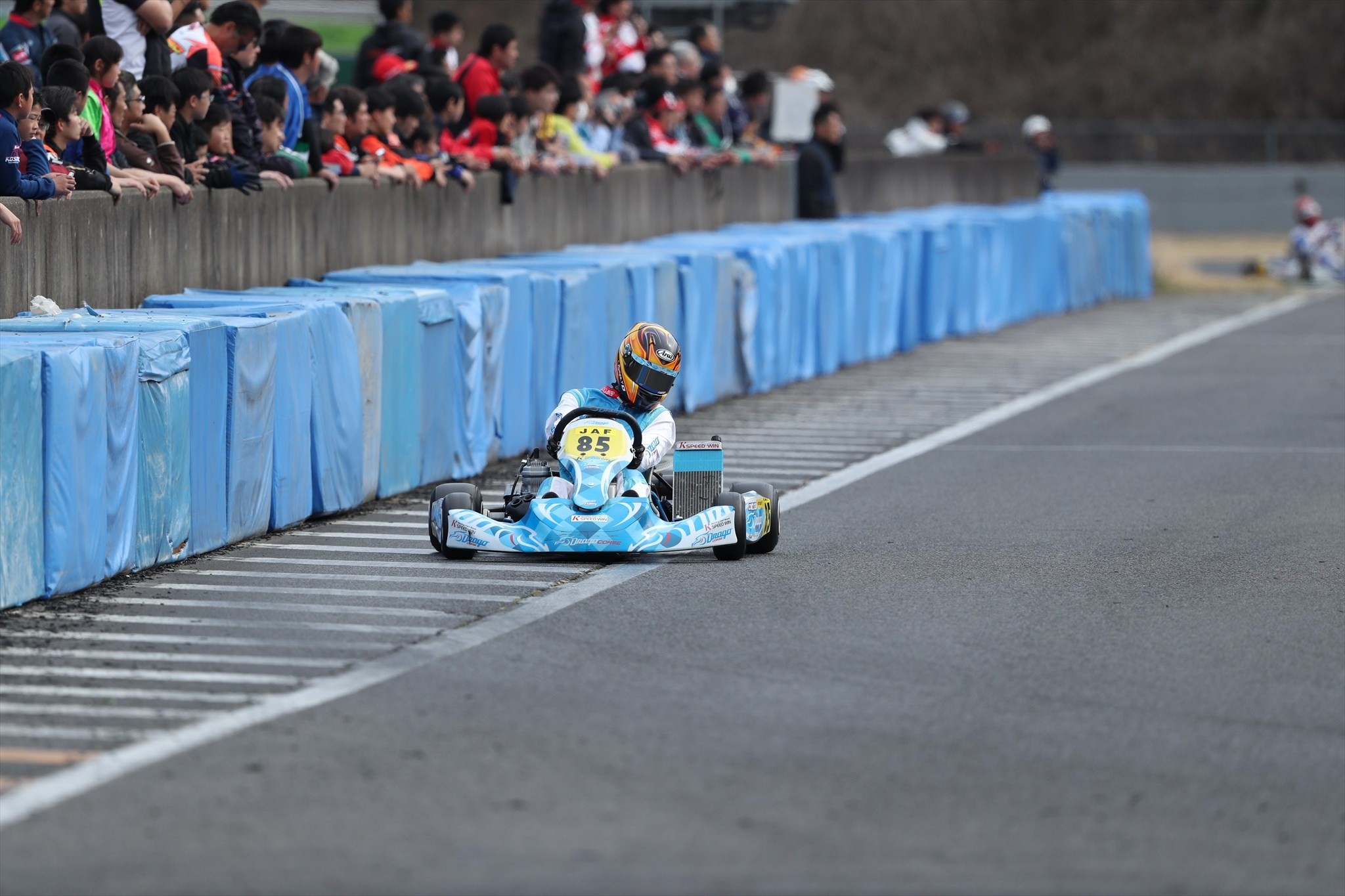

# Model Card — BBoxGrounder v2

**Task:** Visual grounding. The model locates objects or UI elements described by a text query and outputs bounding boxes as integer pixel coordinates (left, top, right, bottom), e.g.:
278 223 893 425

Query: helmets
614 321 681 410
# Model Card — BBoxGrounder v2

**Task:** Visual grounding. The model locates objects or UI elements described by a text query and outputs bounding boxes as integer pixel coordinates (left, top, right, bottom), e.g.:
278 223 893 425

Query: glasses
197 94 215 103
250 38 258 48
38 120 50 131
127 95 146 104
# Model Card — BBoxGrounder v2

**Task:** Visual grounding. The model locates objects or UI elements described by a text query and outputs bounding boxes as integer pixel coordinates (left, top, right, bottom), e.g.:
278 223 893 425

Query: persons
1287 196 1342 265
0 0 1062 247
534 322 682 500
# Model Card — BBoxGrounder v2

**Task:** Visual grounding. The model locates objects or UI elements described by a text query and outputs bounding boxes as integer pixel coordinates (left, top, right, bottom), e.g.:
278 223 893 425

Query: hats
40 98 58 124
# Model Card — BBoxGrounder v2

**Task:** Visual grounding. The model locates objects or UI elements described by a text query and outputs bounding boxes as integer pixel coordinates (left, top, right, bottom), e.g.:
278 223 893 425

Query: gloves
546 435 560 460
626 444 646 470
